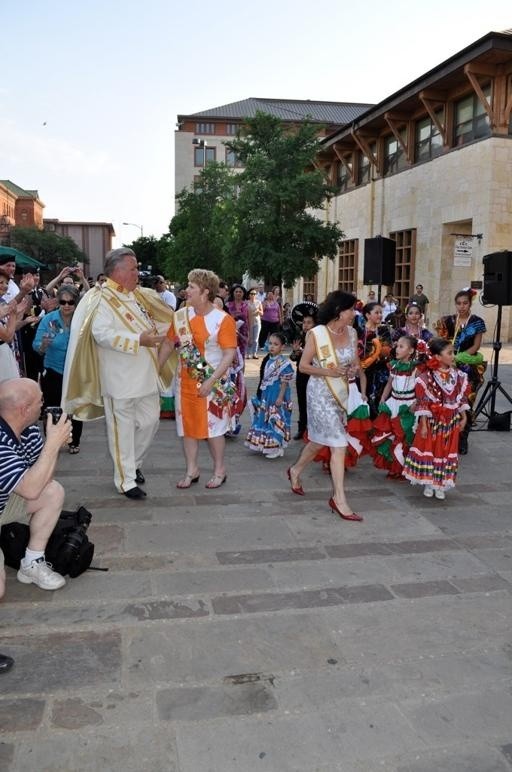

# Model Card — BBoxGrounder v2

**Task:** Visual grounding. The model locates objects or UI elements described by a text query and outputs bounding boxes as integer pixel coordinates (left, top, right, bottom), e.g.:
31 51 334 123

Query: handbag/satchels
1 509 94 579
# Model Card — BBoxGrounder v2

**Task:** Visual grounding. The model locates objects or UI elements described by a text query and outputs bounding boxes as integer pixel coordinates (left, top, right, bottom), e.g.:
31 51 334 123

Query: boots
292 420 307 441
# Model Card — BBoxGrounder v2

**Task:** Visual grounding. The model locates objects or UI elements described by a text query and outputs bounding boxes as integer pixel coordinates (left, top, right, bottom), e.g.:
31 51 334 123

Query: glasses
59 299 76 306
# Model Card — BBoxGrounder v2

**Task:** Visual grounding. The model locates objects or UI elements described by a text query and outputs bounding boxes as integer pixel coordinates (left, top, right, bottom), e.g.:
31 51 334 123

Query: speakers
363 236 396 287
482 250 512 306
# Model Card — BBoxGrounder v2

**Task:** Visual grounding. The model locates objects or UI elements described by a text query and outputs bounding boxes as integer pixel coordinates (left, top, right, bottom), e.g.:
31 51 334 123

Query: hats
0 254 17 267
24 264 41 274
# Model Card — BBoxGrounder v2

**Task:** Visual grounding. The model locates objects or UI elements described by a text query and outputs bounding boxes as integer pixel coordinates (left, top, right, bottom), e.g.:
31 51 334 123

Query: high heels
329 495 363 521
287 466 304 495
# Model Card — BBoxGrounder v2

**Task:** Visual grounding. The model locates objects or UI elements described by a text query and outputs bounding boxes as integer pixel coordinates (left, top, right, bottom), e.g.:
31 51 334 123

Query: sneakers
17 557 66 592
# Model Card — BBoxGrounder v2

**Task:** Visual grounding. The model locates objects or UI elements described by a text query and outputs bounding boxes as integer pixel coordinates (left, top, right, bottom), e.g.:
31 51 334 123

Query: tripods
473 307 512 421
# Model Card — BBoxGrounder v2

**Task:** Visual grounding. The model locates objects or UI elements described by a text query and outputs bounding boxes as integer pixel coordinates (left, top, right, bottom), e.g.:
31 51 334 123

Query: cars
139 270 151 277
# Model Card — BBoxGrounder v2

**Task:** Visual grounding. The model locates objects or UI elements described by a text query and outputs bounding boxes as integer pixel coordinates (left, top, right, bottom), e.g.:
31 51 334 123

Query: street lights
122 221 145 239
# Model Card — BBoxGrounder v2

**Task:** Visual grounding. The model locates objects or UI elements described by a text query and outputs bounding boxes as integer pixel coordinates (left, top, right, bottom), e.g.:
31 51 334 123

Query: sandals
205 472 227 489
69 444 80 454
176 470 201 489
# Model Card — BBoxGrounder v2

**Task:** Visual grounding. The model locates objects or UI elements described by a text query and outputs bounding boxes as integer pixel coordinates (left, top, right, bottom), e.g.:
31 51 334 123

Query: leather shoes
124 486 147 500
457 438 469 457
135 468 146 484
385 471 410 484
264 447 285 459
322 460 349 474
423 486 434 498
435 488 447 500
0 653 16 673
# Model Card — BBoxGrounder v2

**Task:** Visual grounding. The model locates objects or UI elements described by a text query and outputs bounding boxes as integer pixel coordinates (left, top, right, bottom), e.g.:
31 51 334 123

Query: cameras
68 267 76 272
